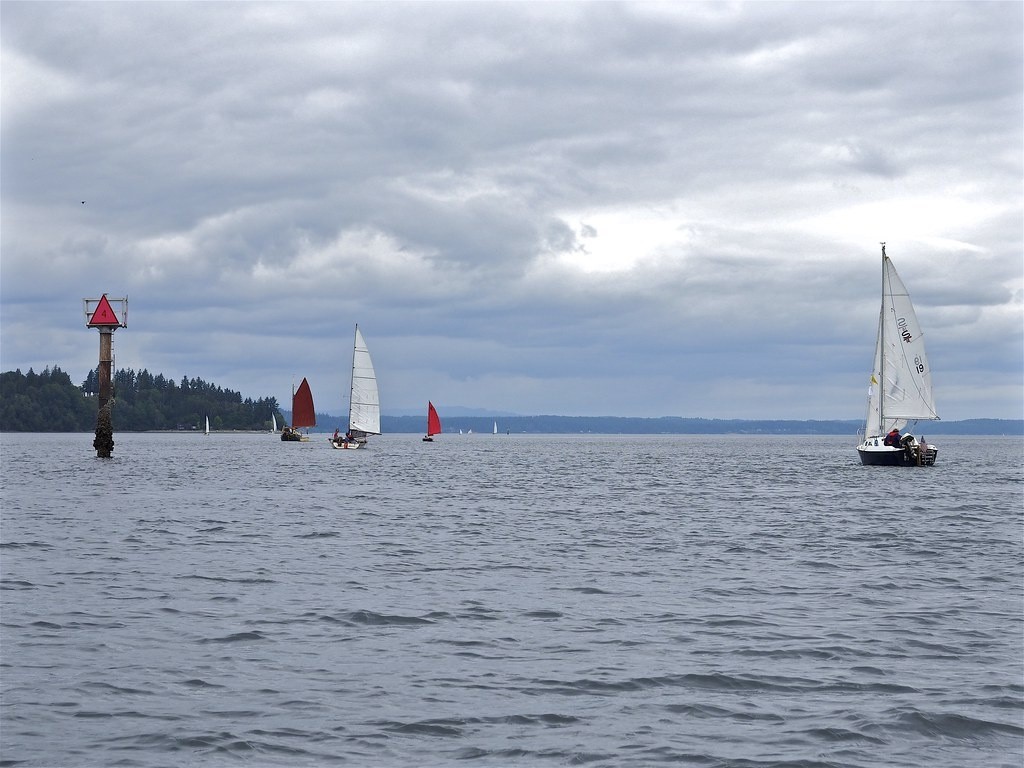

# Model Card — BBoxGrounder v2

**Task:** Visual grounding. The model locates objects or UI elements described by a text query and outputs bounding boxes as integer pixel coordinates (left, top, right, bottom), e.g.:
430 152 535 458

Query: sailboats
856 241 939 465
422 400 442 441
280 377 317 440
328 322 382 450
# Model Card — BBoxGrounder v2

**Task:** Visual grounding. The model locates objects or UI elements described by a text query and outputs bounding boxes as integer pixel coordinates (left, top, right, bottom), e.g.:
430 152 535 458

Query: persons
345 433 350 443
884 428 903 449
333 429 339 439
